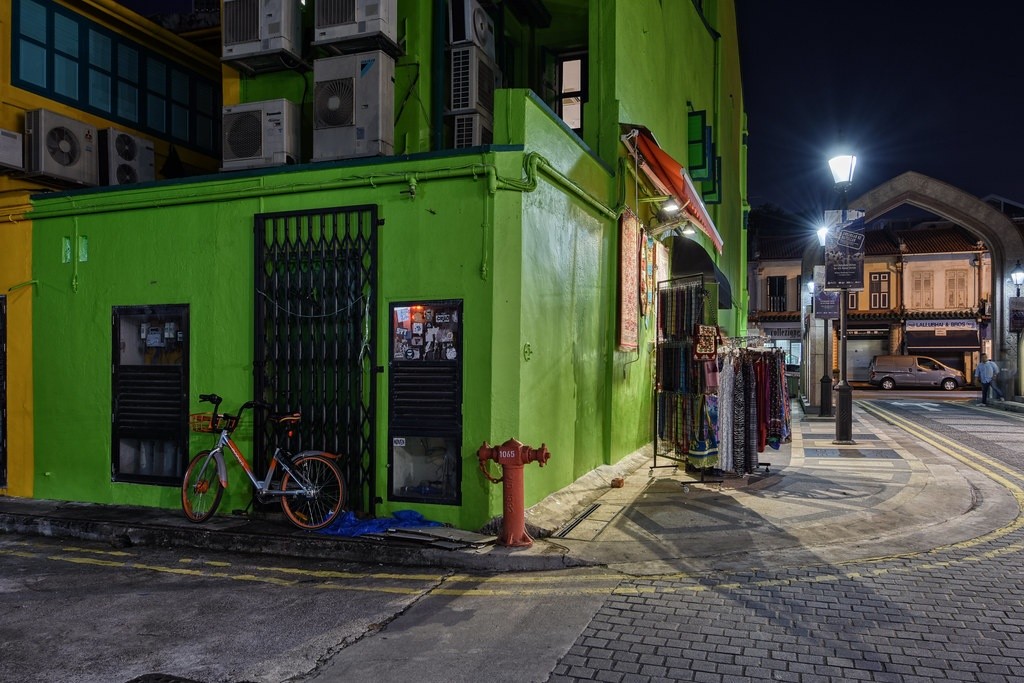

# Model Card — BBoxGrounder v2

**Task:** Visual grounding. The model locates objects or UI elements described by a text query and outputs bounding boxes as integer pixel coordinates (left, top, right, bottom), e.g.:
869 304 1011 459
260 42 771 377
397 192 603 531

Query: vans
867 354 966 391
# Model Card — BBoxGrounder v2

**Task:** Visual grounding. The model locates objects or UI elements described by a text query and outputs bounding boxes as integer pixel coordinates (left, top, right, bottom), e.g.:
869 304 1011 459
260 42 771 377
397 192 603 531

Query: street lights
816 224 835 417
1009 259 1024 404
826 130 859 445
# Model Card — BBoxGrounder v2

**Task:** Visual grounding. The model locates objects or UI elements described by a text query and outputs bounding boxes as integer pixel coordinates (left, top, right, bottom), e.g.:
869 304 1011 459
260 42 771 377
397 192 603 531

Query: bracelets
994 375 997 377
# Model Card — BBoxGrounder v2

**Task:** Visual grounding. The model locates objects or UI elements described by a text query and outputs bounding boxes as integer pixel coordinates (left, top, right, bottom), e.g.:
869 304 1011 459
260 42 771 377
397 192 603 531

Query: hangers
720 335 781 355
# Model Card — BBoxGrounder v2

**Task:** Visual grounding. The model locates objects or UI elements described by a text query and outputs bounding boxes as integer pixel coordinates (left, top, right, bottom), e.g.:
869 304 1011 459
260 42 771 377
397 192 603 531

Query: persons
973 353 1005 407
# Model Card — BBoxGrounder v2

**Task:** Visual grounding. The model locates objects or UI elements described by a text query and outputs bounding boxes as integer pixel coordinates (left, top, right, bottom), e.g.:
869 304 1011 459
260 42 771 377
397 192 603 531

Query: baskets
188 412 237 433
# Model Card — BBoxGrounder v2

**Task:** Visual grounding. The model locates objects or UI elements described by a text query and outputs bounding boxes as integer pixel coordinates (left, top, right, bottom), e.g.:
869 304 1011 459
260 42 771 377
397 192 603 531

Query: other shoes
976 402 986 406
1000 397 1004 402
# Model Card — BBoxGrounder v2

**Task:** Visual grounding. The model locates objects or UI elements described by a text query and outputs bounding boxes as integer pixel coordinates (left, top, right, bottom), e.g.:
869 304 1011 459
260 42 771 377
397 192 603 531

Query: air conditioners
23 107 99 191
453 113 494 149
216 0 302 74
99 124 156 186
309 0 401 51
447 0 497 63
219 98 299 172
449 46 504 120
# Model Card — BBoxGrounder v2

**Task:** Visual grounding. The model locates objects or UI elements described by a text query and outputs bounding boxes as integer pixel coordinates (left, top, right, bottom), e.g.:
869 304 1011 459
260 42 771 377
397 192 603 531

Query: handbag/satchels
708 351 727 373
692 298 722 362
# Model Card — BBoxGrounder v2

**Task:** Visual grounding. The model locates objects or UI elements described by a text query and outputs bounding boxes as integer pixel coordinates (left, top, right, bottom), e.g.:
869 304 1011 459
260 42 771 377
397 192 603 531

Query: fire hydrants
477 437 551 548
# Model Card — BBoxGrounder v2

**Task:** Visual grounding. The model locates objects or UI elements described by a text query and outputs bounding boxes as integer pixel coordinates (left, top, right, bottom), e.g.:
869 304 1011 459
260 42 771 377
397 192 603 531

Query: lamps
639 193 678 211
650 218 695 237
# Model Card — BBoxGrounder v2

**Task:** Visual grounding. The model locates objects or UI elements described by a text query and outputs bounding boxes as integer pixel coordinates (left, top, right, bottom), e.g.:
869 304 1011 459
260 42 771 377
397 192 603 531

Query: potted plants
310 49 396 162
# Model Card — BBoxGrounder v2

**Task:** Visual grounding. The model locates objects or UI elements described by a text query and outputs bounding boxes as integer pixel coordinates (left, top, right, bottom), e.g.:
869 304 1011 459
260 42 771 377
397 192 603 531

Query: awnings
618 122 725 256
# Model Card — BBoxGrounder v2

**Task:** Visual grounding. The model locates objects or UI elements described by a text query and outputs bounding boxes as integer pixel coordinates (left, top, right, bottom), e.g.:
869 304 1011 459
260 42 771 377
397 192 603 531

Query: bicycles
181 393 348 531
